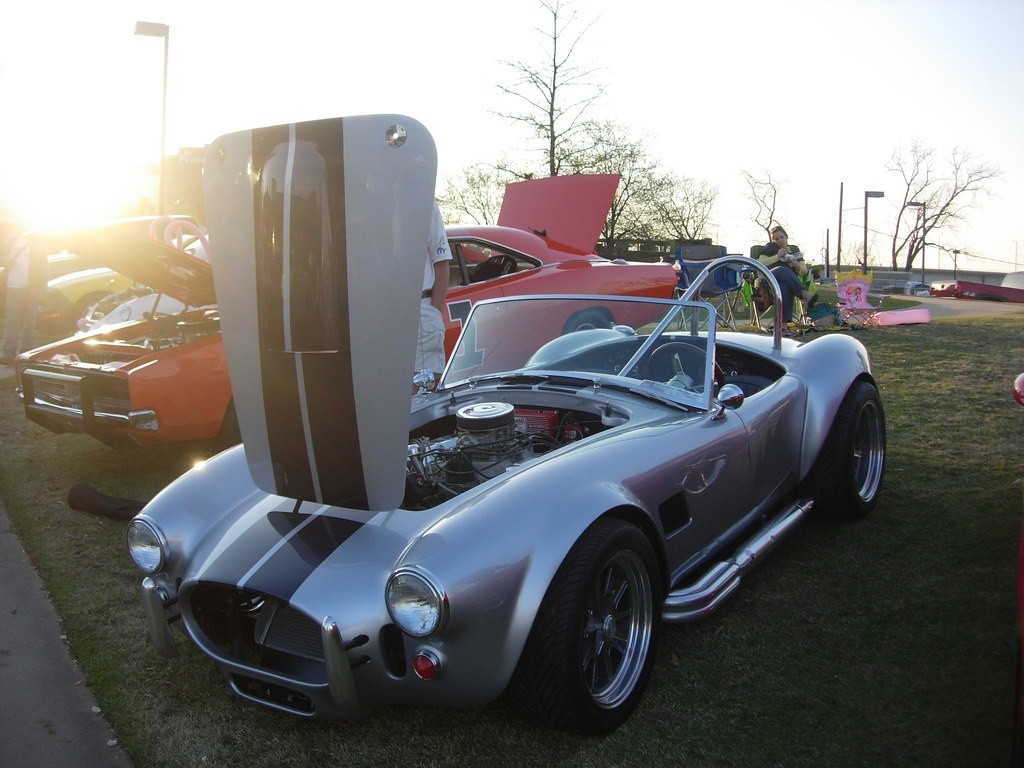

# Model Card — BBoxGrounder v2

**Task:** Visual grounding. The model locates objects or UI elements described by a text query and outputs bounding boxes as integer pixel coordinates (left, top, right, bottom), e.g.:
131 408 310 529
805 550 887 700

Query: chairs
459 255 517 285
833 268 891 330
674 245 743 332
743 244 824 332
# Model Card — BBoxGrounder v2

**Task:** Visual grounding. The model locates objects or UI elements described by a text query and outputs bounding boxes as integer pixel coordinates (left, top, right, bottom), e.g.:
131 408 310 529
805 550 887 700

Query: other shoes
783 329 794 336
808 294 818 307
0 357 16 365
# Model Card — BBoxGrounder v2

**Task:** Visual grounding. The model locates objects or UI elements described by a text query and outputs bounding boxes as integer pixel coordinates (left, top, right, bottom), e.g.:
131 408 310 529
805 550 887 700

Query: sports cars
127 112 889 736
9 170 684 484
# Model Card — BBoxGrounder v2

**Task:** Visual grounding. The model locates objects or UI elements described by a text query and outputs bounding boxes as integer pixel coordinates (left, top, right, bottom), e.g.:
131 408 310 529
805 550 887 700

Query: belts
421 290 432 298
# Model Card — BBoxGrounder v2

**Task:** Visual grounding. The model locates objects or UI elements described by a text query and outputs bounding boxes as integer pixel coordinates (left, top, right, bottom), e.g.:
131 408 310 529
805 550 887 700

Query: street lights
861 191 886 278
907 200 926 289
136 19 172 223
952 250 961 282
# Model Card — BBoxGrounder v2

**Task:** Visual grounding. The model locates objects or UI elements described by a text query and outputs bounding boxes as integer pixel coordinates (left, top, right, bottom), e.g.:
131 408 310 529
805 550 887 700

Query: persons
414 199 453 373
758 226 818 332
0 237 44 364
